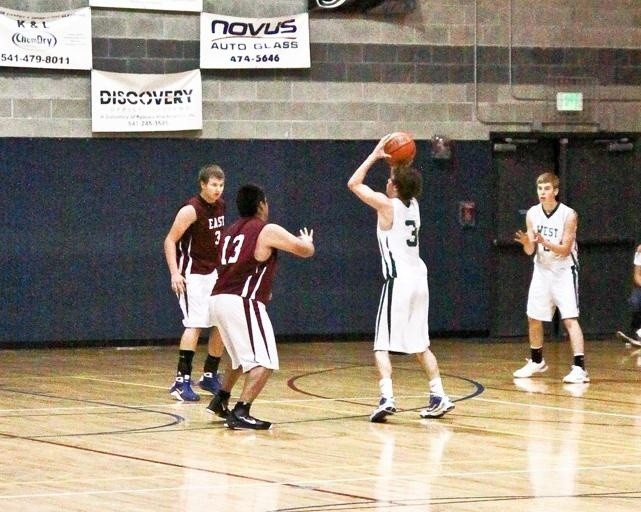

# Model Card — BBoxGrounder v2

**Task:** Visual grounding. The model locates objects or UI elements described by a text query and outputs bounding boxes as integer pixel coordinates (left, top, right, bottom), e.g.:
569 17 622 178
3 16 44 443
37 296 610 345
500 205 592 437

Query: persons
513 171 592 387
345 133 457 424
160 161 228 404
617 241 641 356
209 182 316 431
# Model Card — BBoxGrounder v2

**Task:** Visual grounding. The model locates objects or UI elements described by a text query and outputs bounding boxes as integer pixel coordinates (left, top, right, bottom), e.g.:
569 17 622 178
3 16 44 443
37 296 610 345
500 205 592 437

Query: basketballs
385 132 416 166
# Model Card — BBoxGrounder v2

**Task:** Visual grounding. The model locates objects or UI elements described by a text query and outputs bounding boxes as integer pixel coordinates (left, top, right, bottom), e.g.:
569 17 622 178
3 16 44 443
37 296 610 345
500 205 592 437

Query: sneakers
370 397 395 422
563 365 590 383
616 328 641 345
168 372 272 430
420 393 455 418
513 357 548 377
